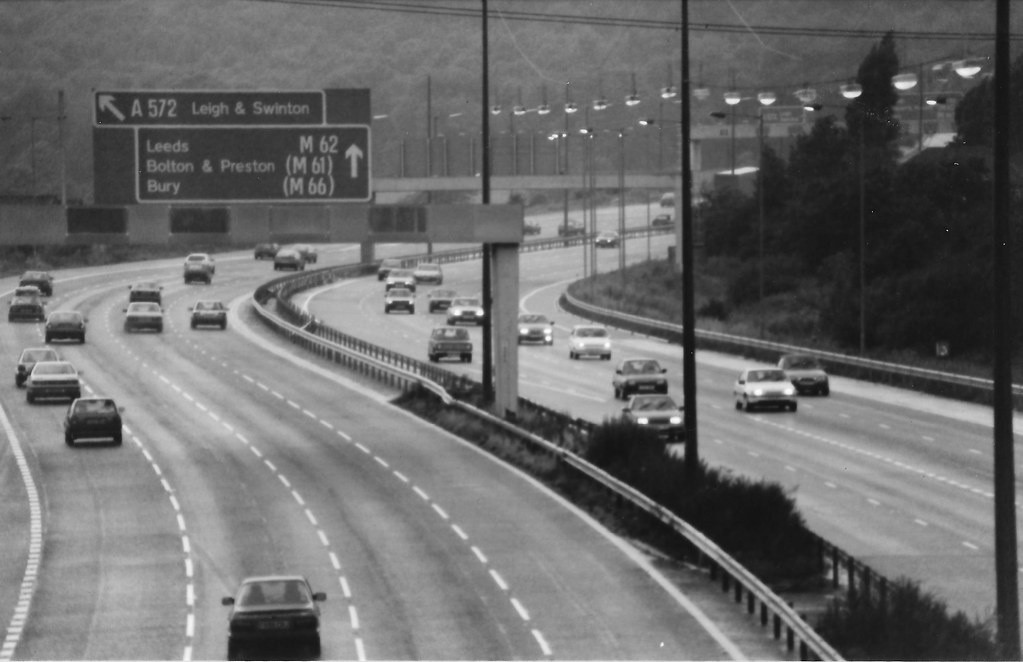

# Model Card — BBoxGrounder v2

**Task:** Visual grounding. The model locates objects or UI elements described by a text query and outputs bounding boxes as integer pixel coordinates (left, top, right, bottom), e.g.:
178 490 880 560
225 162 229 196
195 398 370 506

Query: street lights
710 112 766 340
548 127 652 295
803 105 868 358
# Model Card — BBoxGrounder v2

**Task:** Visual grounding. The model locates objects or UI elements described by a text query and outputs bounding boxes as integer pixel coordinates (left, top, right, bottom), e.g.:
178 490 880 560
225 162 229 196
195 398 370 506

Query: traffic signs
93 91 373 205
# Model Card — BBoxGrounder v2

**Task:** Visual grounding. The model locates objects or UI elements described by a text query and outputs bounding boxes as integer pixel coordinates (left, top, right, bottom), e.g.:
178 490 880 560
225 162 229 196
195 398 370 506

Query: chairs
148 306 155 311
134 307 138 310
150 284 155 288
456 330 463 338
27 353 33 361
284 581 302 600
243 583 265 603
642 363 656 372
104 401 113 409
40 274 45 278
763 372 770 379
749 372 758 380
79 403 88 413
456 300 460 305
469 300 476 306
44 351 52 360
624 363 634 373
213 303 219 310
197 302 204 309
390 291 398 297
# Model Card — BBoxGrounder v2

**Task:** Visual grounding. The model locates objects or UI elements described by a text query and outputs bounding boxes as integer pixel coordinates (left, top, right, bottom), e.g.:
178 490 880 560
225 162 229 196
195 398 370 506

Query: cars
187 301 229 333
64 398 126 445
378 259 484 327
567 325 612 361
613 359 669 399
14 348 84 402
222 575 328 650
124 280 164 334
253 243 316 272
622 395 686 439
518 313 556 347
524 192 676 248
184 253 214 284
6 271 88 346
733 354 829 412
429 328 473 362
